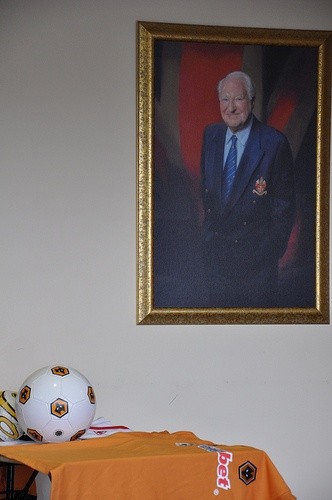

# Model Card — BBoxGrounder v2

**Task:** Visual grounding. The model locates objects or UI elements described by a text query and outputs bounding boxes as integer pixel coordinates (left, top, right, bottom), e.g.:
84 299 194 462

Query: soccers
16 365 96 443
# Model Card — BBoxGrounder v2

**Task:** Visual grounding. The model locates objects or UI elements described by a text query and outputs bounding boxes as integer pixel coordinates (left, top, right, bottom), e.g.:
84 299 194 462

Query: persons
200 70 297 309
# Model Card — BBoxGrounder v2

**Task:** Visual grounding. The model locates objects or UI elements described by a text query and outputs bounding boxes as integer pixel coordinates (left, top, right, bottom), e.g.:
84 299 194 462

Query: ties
221 136 237 210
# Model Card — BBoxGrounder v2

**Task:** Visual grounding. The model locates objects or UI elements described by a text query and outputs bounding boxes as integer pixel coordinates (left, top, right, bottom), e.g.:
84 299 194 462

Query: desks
0 441 297 500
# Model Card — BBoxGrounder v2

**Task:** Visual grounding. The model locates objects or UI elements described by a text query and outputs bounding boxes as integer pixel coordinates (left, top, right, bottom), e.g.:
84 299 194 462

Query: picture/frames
134 19 332 325
